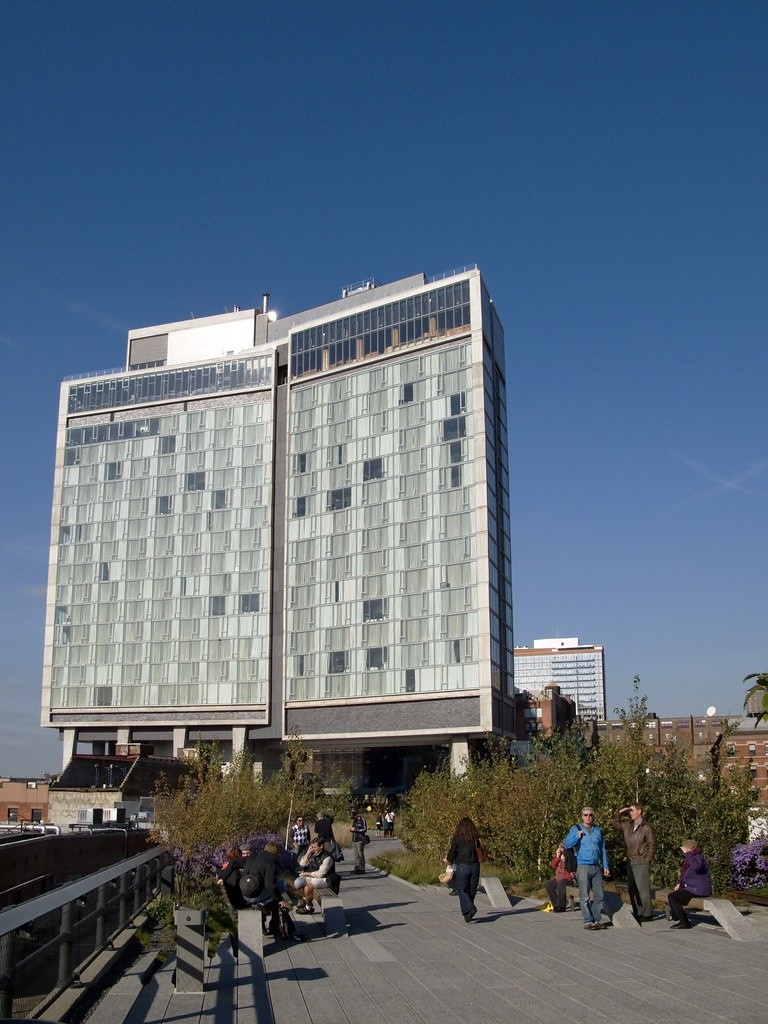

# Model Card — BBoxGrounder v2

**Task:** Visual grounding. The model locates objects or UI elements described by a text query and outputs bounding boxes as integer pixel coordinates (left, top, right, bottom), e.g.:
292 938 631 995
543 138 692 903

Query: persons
611 802 657 922
216 806 397 944
546 842 576 911
562 806 611 930
443 816 483 923
667 840 712 929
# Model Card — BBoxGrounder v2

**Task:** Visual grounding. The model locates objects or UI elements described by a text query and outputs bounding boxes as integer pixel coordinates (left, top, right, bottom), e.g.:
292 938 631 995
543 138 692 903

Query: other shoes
584 922 593 929
449 890 459 896
637 916 653 921
275 934 286 942
592 922 602 929
465 914 471 923
296 907 315 914
297 900 306 907
262 924 269 935
553 907 566 912
468 908 477 920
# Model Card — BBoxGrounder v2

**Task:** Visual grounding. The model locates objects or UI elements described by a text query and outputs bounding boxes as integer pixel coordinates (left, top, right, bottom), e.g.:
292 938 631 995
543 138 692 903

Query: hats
351 807 359 813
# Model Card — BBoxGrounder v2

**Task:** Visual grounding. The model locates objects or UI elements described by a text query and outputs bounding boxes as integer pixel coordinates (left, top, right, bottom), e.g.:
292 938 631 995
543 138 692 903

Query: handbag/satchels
376 822 380 824
361 835 370 845
476 848 487 863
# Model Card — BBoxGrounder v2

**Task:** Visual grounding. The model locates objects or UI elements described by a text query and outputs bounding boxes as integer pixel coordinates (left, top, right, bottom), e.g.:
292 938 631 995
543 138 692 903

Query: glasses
583 813 593 816
297 820 303 821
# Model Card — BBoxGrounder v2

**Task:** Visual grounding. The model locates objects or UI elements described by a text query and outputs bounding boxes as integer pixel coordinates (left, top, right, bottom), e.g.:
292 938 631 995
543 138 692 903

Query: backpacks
564 824 583 873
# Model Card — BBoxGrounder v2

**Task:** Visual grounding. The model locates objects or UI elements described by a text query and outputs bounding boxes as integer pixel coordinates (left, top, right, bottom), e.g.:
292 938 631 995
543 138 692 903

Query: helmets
240 875 259 896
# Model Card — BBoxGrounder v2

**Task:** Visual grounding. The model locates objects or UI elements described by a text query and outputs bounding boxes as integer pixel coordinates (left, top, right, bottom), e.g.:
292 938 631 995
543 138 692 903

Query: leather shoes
669 916 678 920
671 922 691 929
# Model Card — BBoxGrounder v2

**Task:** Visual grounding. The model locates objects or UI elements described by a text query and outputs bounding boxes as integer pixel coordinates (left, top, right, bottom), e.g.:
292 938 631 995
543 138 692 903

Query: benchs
478 877 512 908
566 885 641 929
650 887 764 942
313 888 349 940
